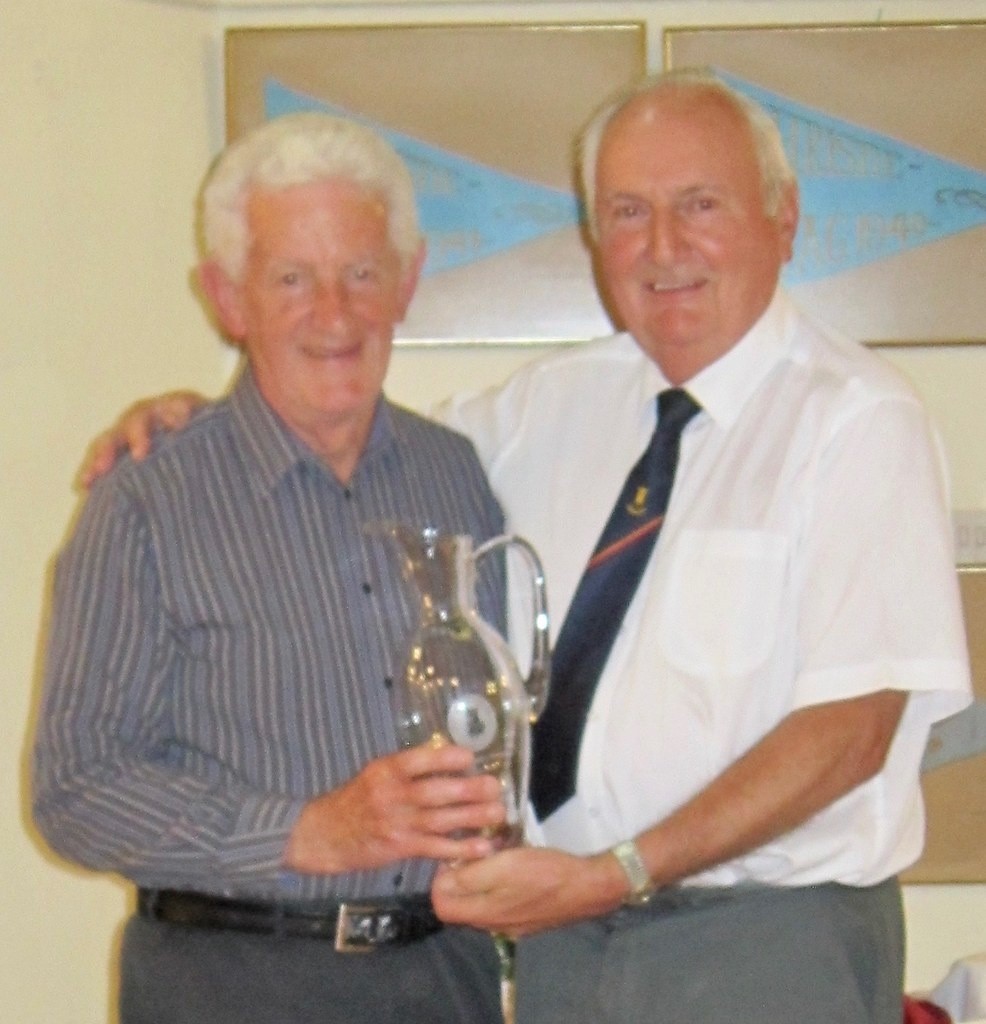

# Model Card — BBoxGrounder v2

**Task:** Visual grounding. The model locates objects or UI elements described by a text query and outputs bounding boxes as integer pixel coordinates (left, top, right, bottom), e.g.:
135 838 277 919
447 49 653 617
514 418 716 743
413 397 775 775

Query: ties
511 388 707 826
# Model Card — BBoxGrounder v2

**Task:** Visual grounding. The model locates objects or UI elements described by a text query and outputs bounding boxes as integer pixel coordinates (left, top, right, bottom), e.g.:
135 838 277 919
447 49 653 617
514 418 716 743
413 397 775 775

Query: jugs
363 510 551 866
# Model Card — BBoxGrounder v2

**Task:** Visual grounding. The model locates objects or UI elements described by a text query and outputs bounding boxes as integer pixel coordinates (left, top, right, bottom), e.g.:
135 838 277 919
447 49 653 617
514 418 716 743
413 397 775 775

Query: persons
78 74 974 1023
28 77 511 1024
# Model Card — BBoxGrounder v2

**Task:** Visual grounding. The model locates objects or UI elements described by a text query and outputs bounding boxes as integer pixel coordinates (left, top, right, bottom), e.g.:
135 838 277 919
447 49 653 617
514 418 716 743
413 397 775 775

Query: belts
132 875 452 955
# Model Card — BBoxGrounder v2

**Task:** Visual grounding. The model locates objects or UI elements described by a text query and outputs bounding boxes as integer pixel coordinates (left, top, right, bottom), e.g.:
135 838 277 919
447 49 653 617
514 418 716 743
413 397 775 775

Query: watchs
606 839 659 907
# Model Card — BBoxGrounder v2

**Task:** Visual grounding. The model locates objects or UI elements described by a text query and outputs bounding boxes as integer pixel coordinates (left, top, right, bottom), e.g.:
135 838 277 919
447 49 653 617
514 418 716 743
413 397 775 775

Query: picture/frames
221 18 646 348
660 18 985 348
890 569 986 886
